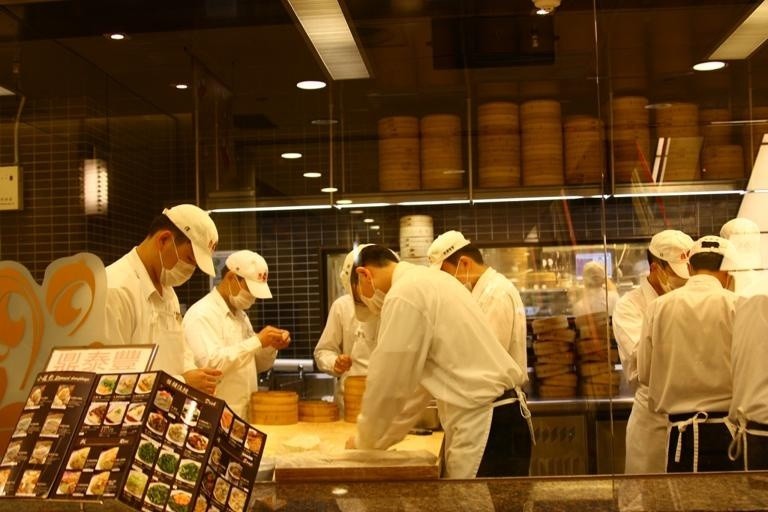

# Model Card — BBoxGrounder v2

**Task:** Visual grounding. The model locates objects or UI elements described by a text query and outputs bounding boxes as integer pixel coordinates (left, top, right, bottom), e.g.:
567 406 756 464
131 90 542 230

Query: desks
247 418 444 479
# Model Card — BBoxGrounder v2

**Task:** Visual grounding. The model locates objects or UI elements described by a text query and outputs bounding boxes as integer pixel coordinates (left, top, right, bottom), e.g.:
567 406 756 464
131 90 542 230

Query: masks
159 240 196 288
228 273 256 311
357 272 387 317
651 263 689 293
453 257 472 292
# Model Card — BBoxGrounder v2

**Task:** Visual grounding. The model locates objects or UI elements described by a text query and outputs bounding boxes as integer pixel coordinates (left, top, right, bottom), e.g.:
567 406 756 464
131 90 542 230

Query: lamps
82 144 110 217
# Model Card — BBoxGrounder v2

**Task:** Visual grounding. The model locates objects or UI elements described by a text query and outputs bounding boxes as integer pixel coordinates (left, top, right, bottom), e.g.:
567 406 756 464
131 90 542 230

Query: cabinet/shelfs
516 397 636 475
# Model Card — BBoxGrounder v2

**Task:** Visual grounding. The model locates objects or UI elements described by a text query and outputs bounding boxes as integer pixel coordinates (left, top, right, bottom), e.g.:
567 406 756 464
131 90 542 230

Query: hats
340 244 379 322
224 250 274 299
583 261 605 285
649 230 695 279
689 235 737 292
427 230 472 271
162 204 219 279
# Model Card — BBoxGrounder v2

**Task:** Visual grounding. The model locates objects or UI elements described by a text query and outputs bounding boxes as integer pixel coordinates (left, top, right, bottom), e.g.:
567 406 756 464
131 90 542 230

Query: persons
314 244 395 420
728 264 768 472
613 230 695 474
569 262 621 316
100 204 221 397
638 235 732 472
180 250 291 423
334 245 534 479
427 230 529 393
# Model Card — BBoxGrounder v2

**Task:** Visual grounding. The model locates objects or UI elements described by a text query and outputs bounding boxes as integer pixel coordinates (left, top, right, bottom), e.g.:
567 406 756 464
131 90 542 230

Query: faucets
299 363 312 400
266 369 272 390
